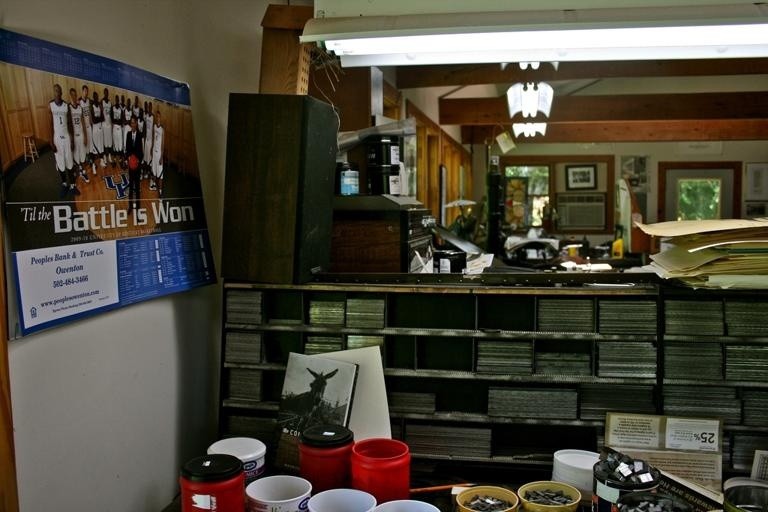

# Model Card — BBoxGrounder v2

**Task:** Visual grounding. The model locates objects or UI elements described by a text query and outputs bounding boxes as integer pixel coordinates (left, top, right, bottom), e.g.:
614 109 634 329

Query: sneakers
127 207 132 214
100 154 113 167
81 170 90 183
93 163 96 175
140 172 149 180
61 186 68 197
70 185 81 194
149 185 157 191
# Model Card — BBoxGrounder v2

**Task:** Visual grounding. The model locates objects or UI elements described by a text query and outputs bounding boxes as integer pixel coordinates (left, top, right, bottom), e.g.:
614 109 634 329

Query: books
646 463 725 512
219 217 767 472
266 351 360 478
749 449 768 482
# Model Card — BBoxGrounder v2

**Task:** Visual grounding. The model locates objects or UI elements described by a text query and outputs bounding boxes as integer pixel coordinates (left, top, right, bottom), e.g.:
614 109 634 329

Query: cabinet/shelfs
212 276 768 479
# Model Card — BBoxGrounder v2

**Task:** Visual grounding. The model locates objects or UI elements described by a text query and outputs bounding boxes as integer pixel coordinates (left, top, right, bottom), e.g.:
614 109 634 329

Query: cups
456 447 662 512
244 474 442 512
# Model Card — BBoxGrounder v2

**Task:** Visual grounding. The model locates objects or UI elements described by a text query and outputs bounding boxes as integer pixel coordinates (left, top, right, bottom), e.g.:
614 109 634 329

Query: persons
45 82 165 216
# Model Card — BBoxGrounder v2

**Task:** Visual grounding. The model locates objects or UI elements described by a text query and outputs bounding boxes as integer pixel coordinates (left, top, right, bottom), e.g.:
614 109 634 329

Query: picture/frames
566 164 597 189
438 164 447 227
744 162 768 202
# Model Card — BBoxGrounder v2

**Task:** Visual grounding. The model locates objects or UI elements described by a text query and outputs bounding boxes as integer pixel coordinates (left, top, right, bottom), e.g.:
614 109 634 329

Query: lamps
299 2 768 68
500 61 560 138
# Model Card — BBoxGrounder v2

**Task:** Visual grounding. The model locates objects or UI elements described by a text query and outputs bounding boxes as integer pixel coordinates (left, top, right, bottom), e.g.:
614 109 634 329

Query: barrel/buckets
552 449 601 512
517 480 582 512
457 485 519 512
178 453 244 512
244 475 312 512
591 458 660 512
375 500 439 512
306 489 377 512
722 477 768 512
207 437 268 484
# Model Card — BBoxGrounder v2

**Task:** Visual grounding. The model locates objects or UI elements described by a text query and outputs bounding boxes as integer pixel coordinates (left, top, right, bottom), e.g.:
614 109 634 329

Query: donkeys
281 367 338 436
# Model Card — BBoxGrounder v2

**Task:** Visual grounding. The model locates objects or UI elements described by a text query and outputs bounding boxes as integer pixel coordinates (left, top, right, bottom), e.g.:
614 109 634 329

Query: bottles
180 436 267 512
